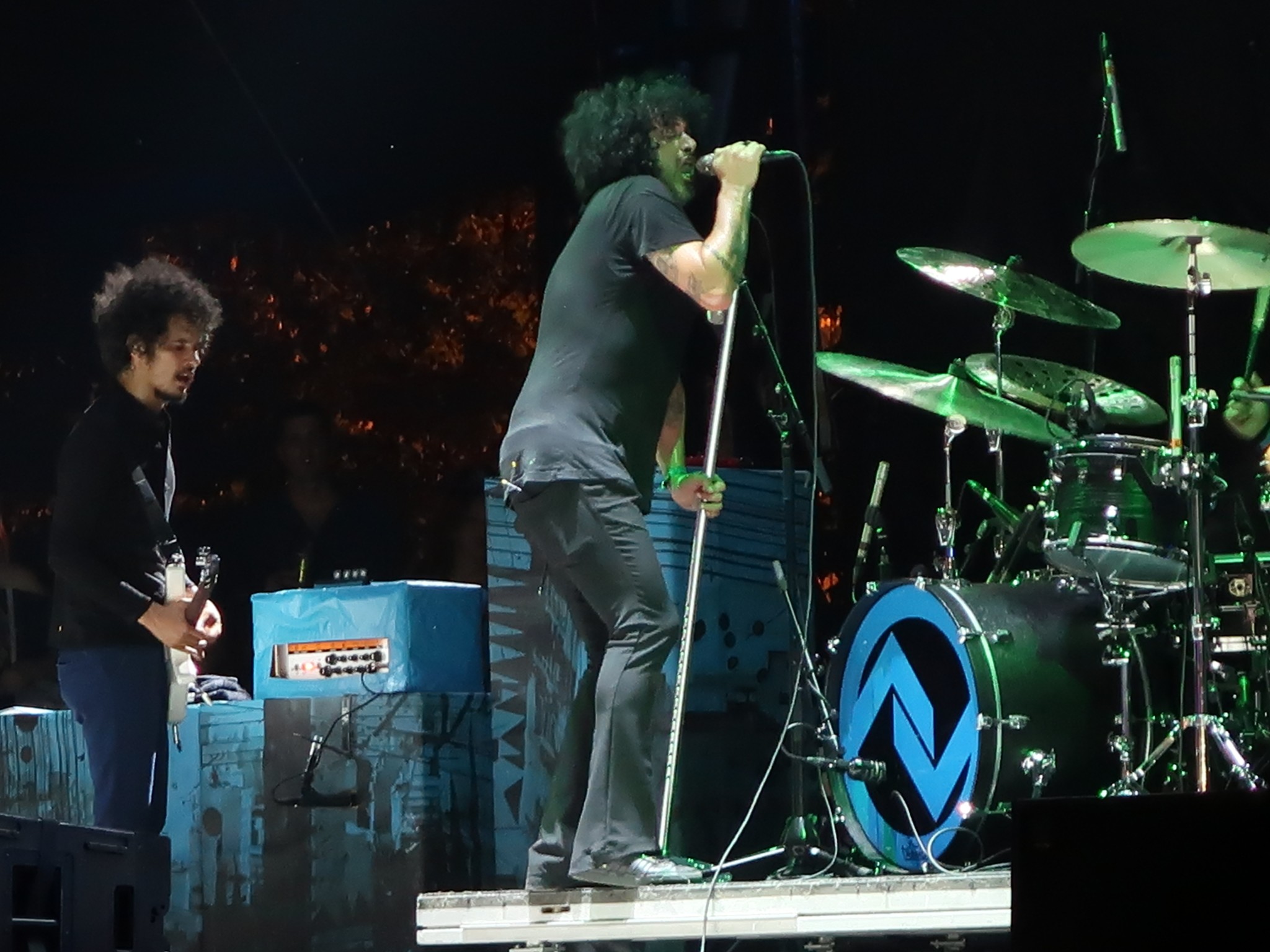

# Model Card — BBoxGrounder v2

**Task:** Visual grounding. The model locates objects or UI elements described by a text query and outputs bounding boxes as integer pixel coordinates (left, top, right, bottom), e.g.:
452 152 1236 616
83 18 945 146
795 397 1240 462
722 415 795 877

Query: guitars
167 546 222 725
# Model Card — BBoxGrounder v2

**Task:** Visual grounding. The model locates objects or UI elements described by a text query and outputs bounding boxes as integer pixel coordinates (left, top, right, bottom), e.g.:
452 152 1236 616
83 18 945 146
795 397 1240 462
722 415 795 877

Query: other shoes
526 876 583 892
571 852 703 885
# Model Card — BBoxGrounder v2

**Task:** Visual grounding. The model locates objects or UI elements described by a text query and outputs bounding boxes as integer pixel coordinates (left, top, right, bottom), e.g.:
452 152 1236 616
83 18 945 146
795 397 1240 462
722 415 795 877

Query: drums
1041 435 1230 583
821 582 1152 874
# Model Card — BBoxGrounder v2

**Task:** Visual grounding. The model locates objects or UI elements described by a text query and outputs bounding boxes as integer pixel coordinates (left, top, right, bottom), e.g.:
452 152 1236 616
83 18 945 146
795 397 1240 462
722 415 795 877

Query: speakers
1010 794 1270 952
0 813 172 952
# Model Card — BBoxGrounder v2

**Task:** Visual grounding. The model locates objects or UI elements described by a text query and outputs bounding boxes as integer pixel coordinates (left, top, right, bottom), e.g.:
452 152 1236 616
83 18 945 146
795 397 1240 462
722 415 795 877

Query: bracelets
662 474 671 491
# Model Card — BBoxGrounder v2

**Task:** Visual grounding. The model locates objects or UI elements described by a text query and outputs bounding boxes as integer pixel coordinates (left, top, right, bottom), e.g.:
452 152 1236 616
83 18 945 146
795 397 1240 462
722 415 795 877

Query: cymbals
892 250 1122 329
958 356 1168 426
813 355 1084 452
1074 217 1268 291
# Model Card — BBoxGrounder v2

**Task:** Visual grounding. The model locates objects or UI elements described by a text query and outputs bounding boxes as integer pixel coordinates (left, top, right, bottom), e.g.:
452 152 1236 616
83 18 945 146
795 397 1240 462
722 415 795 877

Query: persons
499 68 764 890
50 255 224 833
223 399 386 595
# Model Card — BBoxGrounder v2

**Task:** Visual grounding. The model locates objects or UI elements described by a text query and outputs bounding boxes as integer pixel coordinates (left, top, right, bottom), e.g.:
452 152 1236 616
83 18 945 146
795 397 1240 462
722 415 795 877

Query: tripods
702 562 874 880
1103 239 1262 794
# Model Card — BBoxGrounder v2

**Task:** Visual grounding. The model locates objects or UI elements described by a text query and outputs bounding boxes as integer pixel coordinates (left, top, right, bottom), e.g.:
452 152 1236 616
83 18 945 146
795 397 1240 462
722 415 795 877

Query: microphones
696 150 796 177
279 789 365 808
1250 287 1269 335
966 479 1022 534
806 757 888 786
853 461 890 585
1101 32 1127 152
1082 383 1108 432
1168 355 1183 487
